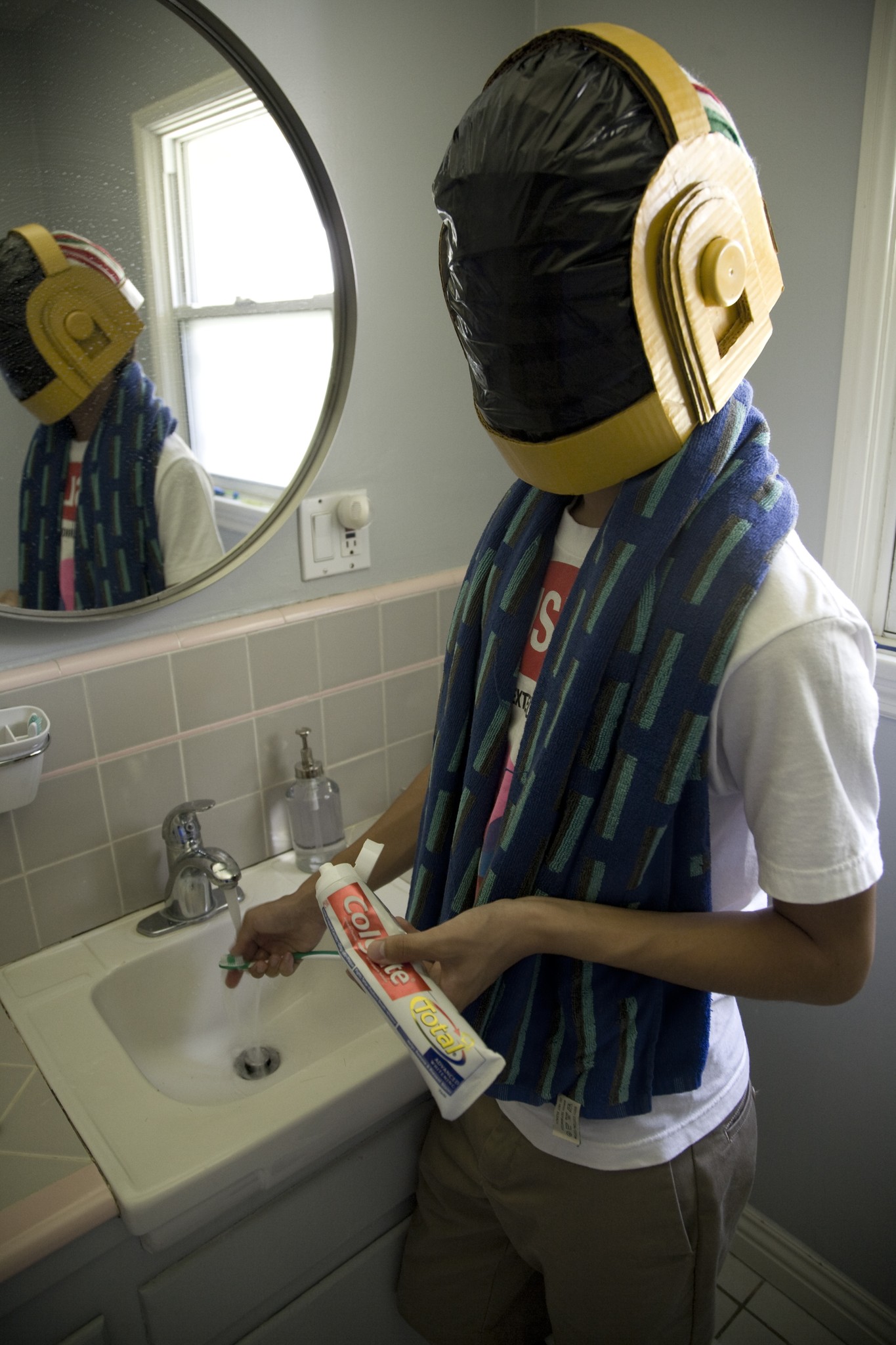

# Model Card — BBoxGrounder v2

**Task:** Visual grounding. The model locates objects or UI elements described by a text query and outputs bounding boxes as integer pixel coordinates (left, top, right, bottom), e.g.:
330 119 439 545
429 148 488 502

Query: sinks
1 837 458 1236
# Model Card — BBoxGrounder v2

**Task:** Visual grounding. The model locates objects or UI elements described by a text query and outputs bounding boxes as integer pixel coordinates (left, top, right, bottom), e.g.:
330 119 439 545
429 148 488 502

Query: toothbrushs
217 943 345 971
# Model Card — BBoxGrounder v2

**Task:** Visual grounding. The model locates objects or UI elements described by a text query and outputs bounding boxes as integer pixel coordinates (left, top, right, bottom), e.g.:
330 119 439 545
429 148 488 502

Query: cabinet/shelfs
0 1093 431 1345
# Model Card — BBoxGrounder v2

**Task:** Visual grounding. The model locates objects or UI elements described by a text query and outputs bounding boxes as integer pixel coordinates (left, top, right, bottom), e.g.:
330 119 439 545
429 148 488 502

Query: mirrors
0 0 360 622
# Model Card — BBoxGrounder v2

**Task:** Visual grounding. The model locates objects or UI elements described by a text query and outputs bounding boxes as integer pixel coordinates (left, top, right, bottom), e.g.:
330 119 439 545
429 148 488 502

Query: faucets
136 799 245 939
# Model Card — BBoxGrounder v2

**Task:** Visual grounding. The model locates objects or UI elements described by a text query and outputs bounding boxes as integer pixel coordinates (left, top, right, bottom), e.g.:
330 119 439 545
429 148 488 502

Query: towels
403 375 798 1118
15 359 178 612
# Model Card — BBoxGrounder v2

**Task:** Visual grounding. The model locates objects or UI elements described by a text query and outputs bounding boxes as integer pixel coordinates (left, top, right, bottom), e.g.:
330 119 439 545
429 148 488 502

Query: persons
222 21 883 1344
1 224 222 613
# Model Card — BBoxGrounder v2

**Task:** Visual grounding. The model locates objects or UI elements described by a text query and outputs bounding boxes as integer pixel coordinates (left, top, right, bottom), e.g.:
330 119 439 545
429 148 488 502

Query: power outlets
299 488 372 582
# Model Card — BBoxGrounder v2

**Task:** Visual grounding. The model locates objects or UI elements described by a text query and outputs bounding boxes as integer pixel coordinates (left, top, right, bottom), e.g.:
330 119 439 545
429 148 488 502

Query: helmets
427 11 788 512
1 223 147 433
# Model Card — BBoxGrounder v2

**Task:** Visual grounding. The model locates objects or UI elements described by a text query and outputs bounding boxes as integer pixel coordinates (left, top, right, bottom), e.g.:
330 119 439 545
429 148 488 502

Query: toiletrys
279 727 350 878
312 838 507 1123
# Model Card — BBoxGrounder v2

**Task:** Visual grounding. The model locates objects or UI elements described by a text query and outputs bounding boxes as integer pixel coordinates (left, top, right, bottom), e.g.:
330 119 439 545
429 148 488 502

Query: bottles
285 727 346 873
